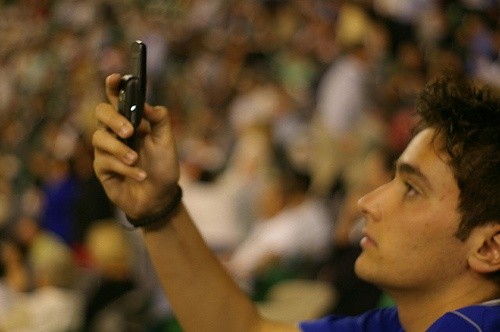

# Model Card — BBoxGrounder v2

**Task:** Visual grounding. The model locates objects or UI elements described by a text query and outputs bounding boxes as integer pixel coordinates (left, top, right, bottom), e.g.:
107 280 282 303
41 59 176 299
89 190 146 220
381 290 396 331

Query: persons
91 74 496 331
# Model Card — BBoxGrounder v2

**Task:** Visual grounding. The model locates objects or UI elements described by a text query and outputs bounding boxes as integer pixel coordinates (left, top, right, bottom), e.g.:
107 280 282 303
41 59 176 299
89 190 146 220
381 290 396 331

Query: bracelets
114 185 182 228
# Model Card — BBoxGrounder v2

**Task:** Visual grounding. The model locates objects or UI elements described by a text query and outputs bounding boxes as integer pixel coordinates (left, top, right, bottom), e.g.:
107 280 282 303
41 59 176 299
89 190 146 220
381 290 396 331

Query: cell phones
118 40 146 147
